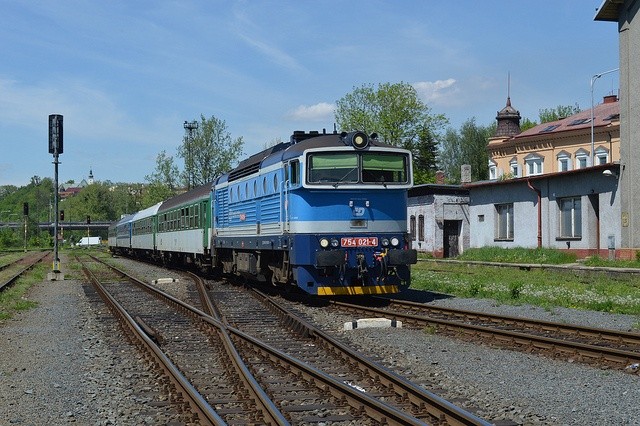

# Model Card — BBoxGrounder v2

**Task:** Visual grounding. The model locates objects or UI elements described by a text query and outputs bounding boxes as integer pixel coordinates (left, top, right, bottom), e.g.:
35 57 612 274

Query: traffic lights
23 202 28 215
59 210 63 220
48 114 63 154
86 215 89 223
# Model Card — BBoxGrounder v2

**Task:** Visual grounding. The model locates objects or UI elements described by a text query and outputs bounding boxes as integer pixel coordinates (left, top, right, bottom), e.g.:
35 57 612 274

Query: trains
108 123 417 300
